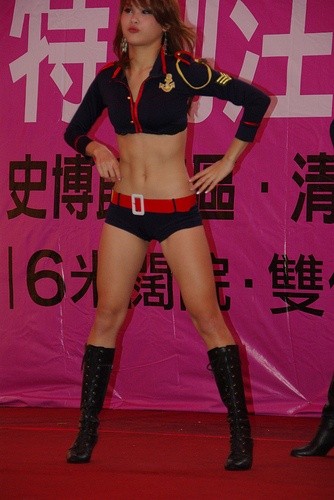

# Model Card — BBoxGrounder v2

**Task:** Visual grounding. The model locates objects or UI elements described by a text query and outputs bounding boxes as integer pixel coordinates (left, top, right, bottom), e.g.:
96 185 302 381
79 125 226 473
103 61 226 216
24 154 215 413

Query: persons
290 377 334 457
64 0 271 471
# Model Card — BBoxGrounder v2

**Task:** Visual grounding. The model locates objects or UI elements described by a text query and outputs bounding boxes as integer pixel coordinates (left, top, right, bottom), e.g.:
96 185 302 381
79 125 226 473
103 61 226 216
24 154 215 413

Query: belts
110 190 197 215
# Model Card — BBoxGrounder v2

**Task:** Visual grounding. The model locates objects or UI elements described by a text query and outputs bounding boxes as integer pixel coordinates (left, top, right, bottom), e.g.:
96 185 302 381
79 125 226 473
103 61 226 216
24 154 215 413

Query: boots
291 374 334 456
207 345 254 471
66 341 116 463
